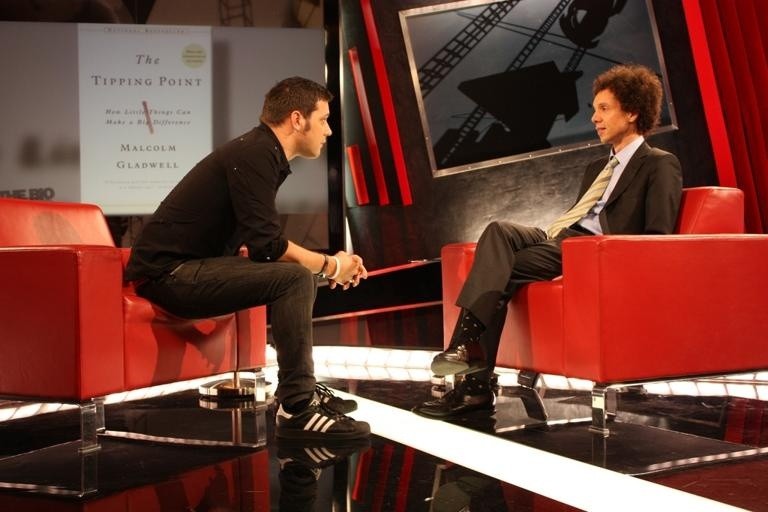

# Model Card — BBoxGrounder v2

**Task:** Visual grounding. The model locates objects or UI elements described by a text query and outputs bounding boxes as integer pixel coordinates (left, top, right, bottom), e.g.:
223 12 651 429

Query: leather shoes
430 343 489 376
420 384 493 416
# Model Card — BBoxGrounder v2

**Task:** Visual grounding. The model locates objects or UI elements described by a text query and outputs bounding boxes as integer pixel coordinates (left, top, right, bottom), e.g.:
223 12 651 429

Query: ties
549 158 618 239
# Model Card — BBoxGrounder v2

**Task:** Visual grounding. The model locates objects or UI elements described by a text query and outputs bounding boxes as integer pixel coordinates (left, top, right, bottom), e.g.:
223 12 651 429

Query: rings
348 279 354 284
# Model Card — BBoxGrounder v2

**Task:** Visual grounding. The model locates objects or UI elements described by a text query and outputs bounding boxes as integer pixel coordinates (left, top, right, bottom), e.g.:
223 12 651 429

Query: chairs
2 193 271 453
440 186 768 435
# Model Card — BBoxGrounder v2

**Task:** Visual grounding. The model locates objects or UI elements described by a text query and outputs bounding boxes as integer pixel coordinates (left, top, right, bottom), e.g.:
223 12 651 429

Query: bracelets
327 256 340 279
314 255 328 279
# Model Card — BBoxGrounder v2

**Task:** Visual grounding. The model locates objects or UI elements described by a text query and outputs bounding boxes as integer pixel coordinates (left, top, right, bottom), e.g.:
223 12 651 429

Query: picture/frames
399 2 679 179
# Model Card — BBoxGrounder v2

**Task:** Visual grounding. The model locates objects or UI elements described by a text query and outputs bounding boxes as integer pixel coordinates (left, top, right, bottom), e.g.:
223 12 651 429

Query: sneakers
272 382 357 417
274 392 369 442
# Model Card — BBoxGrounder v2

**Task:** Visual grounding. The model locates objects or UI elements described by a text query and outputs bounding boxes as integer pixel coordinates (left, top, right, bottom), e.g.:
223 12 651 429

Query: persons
124 77 368 439
412 67 682 420
